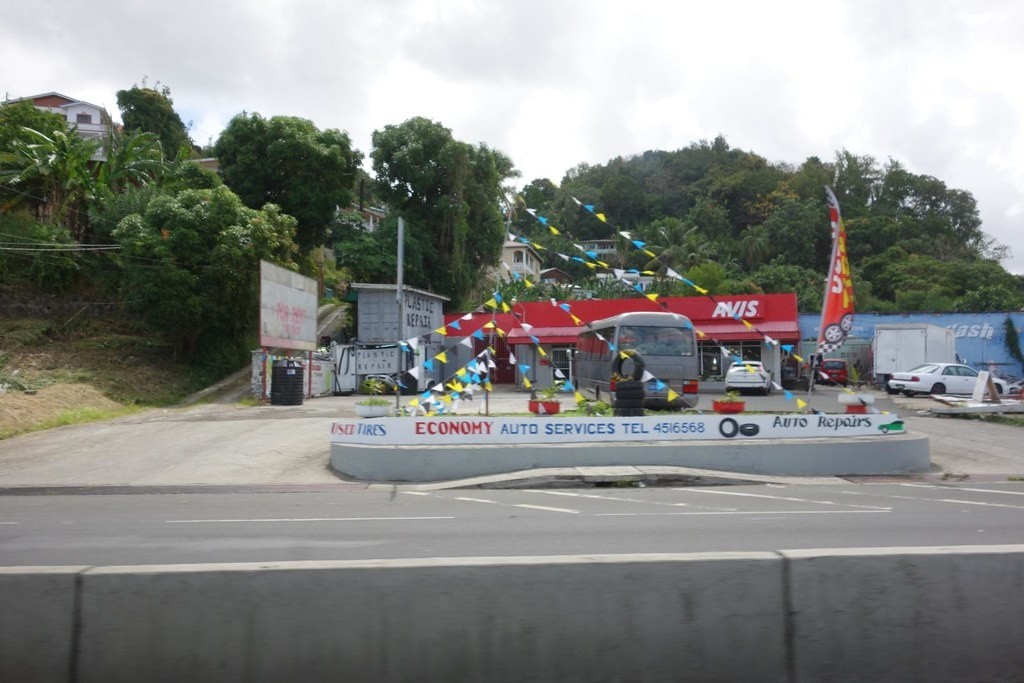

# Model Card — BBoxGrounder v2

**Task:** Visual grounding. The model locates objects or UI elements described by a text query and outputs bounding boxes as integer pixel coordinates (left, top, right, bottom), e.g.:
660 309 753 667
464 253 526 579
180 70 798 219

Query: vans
780 358 813 392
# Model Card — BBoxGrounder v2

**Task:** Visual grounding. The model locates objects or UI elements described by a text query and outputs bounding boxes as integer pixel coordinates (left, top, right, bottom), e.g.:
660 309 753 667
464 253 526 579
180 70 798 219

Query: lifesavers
612 381 646 416
612 349 645 382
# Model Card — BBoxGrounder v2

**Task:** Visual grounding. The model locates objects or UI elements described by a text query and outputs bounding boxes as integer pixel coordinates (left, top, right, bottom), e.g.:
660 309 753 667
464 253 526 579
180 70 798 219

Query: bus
573 312 700 413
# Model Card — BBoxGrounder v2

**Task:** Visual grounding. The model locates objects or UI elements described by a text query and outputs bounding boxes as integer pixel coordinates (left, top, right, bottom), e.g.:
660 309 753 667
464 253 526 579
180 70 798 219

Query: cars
888 363 1009 398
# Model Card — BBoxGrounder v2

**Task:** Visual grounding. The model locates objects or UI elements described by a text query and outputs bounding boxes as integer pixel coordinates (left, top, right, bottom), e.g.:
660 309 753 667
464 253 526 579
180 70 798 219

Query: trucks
873 323 967 395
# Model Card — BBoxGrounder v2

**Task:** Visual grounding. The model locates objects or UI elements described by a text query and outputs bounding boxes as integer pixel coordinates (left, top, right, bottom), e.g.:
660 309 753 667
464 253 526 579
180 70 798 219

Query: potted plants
711 389 747 415
843 365 867 413
351 370 398 416
528 381 566 416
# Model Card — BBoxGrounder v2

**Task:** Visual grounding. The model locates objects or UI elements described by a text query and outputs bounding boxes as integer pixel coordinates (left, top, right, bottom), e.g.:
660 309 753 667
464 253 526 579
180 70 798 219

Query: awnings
508 321 802 345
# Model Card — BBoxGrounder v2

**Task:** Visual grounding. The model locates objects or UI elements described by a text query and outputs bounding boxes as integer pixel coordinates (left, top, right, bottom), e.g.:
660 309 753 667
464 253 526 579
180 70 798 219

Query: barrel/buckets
271 360 304 405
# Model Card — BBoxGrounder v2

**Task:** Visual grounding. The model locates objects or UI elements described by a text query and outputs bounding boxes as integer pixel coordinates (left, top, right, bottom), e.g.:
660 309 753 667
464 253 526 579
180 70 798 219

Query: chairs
945 369 953 374
636 343 690 354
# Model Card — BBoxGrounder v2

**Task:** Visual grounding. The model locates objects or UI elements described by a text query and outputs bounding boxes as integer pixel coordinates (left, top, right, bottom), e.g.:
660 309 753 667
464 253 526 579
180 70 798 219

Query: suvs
818 359 849 388
724 361 772 396
